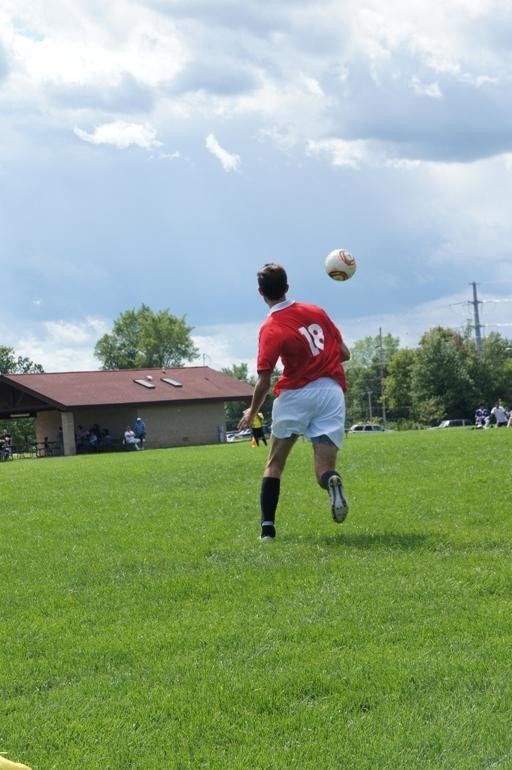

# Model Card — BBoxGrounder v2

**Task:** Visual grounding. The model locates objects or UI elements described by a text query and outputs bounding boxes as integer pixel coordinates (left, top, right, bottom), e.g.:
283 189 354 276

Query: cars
439 419 473 428
349 424 394 433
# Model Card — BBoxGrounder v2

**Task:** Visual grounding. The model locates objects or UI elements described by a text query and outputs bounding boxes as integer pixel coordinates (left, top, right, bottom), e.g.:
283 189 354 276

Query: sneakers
257 525 276 542
328 475 348 523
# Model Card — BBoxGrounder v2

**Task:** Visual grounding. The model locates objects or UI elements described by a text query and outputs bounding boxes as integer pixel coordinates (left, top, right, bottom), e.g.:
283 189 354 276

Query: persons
474 399 512 429
74 423 113 453
44 437 48 448
57 426 64 440
124 417 145 450
251 412 267 447
237 263 351 539
0 429 12 460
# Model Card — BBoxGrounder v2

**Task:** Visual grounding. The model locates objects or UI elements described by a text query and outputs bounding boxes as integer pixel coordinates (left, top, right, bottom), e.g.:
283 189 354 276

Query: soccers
324 249 356 280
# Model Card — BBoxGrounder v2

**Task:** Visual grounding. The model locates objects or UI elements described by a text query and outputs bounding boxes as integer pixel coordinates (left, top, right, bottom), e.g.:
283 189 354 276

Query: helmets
136 418 141 421
